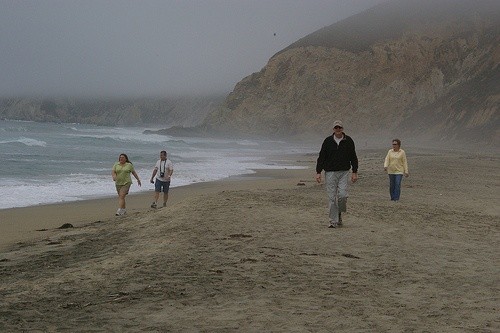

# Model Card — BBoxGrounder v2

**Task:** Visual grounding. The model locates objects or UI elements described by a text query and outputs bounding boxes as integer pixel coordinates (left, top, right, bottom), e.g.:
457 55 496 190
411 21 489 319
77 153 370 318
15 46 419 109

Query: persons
384 139 408 202
150 150 174 208
111 153 143 217
316 120 358 229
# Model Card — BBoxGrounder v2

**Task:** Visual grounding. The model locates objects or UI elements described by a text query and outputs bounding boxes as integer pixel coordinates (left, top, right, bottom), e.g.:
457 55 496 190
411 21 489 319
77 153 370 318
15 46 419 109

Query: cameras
160 172 164 177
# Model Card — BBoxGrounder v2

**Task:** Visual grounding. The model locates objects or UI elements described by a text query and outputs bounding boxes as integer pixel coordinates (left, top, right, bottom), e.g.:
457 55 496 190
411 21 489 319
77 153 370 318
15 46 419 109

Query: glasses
334 126 342 129
392 144 398 146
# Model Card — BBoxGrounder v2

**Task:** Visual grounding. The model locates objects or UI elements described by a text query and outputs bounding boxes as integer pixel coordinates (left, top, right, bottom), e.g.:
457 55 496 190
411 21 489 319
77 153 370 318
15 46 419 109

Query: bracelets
168 176 170 178
137 179 140 181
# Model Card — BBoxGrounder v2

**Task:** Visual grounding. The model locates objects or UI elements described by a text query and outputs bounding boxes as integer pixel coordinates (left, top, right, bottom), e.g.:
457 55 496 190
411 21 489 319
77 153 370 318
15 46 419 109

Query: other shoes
391 200 398 203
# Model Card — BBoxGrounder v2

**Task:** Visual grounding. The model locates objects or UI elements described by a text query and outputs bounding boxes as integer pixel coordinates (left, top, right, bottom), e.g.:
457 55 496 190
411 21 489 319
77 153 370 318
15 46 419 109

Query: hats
333 120 343 128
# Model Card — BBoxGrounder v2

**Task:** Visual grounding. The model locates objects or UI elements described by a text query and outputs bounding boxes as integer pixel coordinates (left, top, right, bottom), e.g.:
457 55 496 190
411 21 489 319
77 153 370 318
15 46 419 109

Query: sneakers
337 220 342 225
328 223 336 228
121 208 126 216
116 208 121 215
151 202 157 208
160 203 166 208
